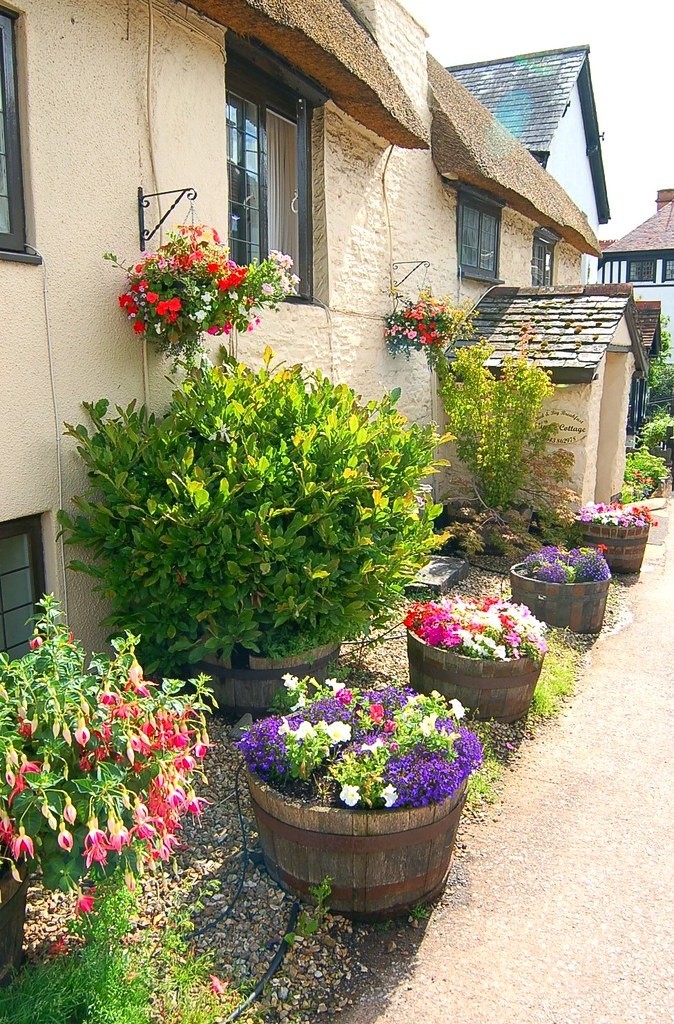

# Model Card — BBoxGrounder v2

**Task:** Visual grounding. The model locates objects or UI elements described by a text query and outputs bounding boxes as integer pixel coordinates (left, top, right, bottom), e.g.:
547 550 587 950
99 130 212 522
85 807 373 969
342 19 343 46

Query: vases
406 629 546 724
573 521 651 574
510 563 613 634
245 762 470 925
0 861 30 990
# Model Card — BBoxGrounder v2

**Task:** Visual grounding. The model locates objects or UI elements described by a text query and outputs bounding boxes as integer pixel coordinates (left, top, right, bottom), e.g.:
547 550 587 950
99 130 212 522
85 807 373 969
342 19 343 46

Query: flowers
523 544 614 583
103 225 301 373
402 591 550 669
233 671 486 810
0 591 218 921
573 502 658 527
382 280 480 363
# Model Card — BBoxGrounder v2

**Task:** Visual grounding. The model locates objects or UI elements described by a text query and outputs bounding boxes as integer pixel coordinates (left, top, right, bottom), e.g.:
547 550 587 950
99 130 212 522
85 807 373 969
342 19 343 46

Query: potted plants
55 344 457 726
425 335 586 563
